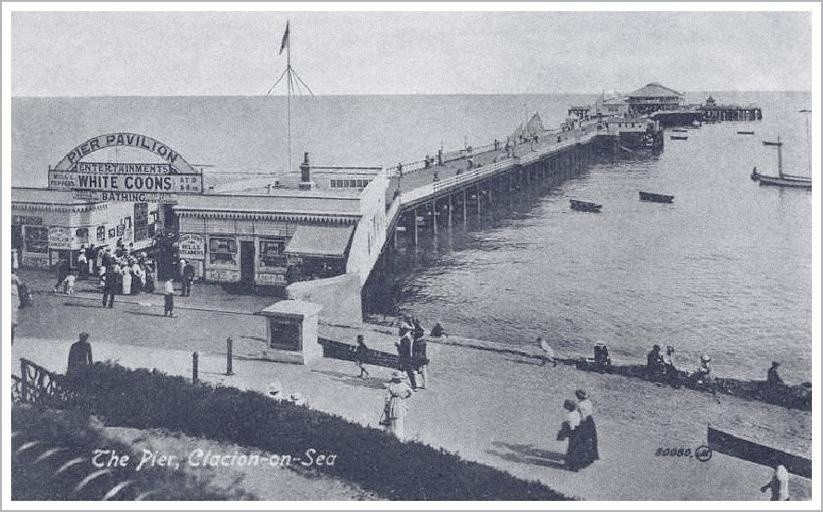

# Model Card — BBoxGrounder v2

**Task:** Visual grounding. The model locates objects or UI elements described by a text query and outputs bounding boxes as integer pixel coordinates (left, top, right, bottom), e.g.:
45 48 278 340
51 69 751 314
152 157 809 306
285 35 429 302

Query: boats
670 127 782 145
750 136 812 188
638 190 675 202
569 198 602 211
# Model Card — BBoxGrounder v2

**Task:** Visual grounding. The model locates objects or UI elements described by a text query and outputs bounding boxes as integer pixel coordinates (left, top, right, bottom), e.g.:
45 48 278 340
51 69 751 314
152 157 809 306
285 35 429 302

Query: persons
535 336 556 368
266 383 281 401
575 390 599 462
52 232 197 319
760 453 790 501
593 341 613 376
289 391 302 404
355 335 368 377
648 344 717 395
767 360 786 394
395 318 448 391
67 331 93 375
393 125 585 201
11 268 22 346
557 399 587 472
383 371 414 447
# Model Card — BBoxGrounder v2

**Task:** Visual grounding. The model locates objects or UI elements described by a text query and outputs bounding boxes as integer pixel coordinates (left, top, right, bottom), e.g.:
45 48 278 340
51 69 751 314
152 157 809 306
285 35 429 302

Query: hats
391 370 407 379
595 341 606 347
700 355 711 361
564 398 576 410
575 389 588 399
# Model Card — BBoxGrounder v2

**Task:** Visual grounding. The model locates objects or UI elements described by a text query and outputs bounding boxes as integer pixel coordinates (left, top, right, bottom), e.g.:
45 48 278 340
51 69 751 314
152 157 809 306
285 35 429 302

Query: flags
279 20 290 54
